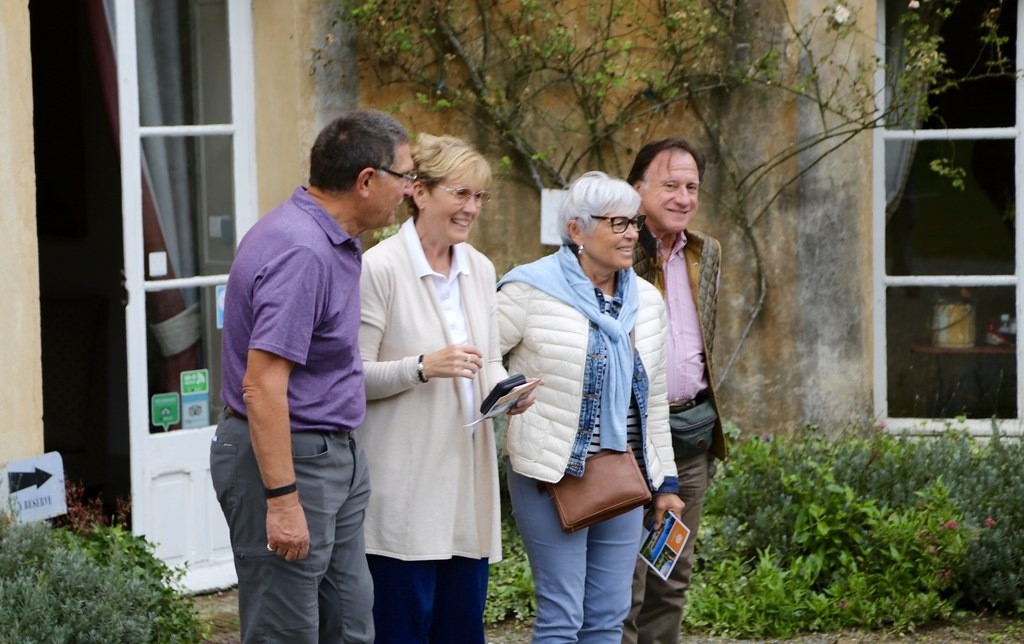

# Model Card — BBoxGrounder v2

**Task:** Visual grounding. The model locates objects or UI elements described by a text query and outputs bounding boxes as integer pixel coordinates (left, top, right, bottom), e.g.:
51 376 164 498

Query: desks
910 337 1017 418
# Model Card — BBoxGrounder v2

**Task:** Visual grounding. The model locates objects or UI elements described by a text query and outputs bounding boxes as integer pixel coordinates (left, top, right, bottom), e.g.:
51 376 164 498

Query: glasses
420 179 491 208
381 166 418 188
590 214 646 233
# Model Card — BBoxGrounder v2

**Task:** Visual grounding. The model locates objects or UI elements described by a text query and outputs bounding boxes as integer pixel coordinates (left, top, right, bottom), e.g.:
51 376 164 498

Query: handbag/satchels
545 442 653 534
669 401 718 460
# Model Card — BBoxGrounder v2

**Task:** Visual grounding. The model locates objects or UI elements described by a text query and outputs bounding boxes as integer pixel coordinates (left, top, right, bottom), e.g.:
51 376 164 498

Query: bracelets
265 483 297 498
418 355 428 383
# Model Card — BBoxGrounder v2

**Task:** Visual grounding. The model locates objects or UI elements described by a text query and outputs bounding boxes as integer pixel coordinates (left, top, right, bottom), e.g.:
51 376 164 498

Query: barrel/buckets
923 300 977 348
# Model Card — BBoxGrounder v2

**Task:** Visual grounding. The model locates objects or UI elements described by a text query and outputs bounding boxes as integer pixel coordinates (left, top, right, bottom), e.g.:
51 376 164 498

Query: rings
468 355 472 362
267 544 276 552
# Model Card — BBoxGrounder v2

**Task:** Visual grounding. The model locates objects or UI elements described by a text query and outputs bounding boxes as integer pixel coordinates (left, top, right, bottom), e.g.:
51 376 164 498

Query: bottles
998 313 1017 343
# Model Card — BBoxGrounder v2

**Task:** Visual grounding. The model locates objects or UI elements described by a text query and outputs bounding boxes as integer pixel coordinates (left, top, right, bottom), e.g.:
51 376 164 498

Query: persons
493 171 686 643
209 108 415 644
359 132 542 644
621 138 726 644
